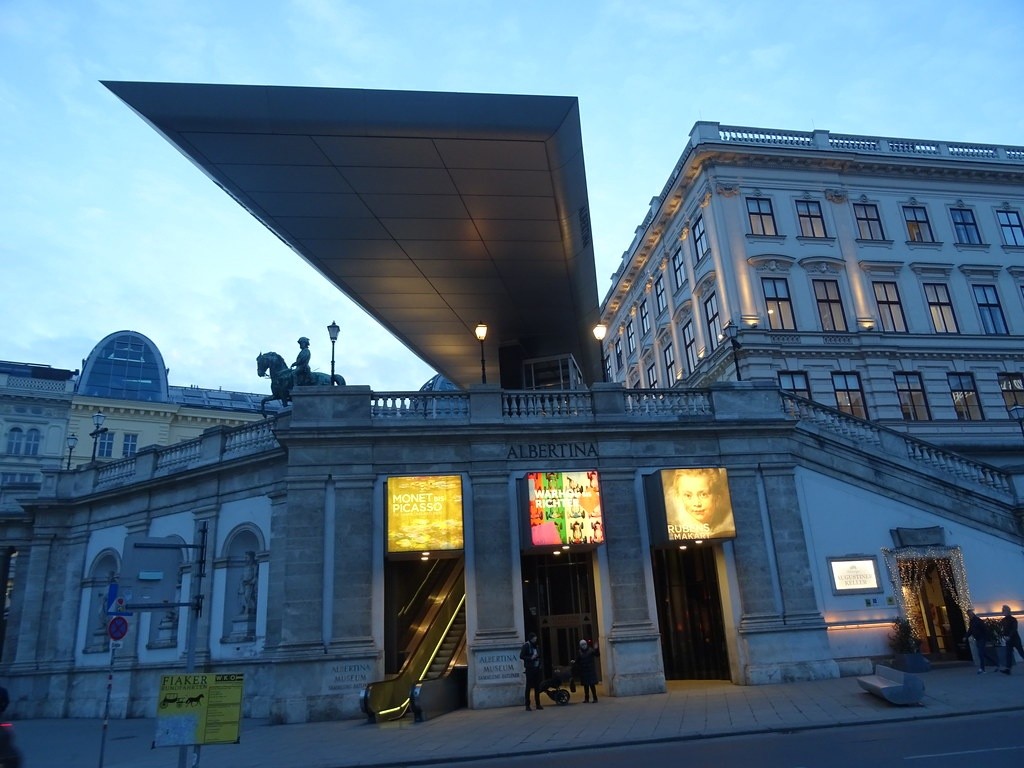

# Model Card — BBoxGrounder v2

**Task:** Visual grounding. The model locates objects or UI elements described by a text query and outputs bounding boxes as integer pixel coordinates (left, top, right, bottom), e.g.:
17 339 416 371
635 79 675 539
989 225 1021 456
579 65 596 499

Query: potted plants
982 618 1016 668
887 616 929 674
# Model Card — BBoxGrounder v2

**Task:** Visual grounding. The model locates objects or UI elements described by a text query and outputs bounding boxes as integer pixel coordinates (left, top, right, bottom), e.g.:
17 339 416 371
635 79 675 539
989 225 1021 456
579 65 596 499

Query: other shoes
1000 669 1010 675
994 665 1000 671
536 706 543 710
977 670 986 674
526 707 531 711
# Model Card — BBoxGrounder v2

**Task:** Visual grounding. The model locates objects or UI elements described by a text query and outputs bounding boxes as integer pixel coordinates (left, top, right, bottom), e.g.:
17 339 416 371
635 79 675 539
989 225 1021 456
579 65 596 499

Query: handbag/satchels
570 678 576 693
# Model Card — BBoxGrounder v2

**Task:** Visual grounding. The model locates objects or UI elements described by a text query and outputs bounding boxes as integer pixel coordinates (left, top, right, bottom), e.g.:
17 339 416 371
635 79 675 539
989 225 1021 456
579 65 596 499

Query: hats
529 632 536 640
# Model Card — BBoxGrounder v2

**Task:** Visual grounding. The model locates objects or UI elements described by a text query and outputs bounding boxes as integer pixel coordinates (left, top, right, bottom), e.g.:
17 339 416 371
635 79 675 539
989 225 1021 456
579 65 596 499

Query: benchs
857 664 925 707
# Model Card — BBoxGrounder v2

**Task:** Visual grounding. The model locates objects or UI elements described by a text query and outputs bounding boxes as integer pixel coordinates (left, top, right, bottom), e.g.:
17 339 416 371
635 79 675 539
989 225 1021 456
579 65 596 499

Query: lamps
752 323 757 329
867 326 873 331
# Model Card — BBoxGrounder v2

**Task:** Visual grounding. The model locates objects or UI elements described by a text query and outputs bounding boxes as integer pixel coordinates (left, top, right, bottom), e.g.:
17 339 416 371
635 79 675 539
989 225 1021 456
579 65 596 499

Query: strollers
542 659 578 706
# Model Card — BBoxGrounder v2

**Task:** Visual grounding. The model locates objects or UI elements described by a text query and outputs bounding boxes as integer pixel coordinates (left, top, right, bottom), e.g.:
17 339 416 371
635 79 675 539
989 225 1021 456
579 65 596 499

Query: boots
592 696 598 703
583 695 589 703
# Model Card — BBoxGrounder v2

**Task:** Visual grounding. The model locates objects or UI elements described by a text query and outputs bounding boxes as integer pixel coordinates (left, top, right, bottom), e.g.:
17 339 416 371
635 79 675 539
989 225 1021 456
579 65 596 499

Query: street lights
88 408 108 462
326 320 341 387
592 320 609 380
475 319 488 385
66 432 79 469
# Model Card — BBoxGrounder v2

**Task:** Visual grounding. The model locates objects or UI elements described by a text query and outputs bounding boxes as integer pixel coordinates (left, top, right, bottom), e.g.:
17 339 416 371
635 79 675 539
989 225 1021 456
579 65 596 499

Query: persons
998 605 1024 674
571 639 599 703
520 632 544 711
287 337 311 401
965 610 1000 674
666 470 735 537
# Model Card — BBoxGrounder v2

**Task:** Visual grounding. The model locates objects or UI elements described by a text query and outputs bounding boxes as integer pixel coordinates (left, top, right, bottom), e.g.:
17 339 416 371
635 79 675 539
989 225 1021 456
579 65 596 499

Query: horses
186 694 204 708
257 352 346 418
